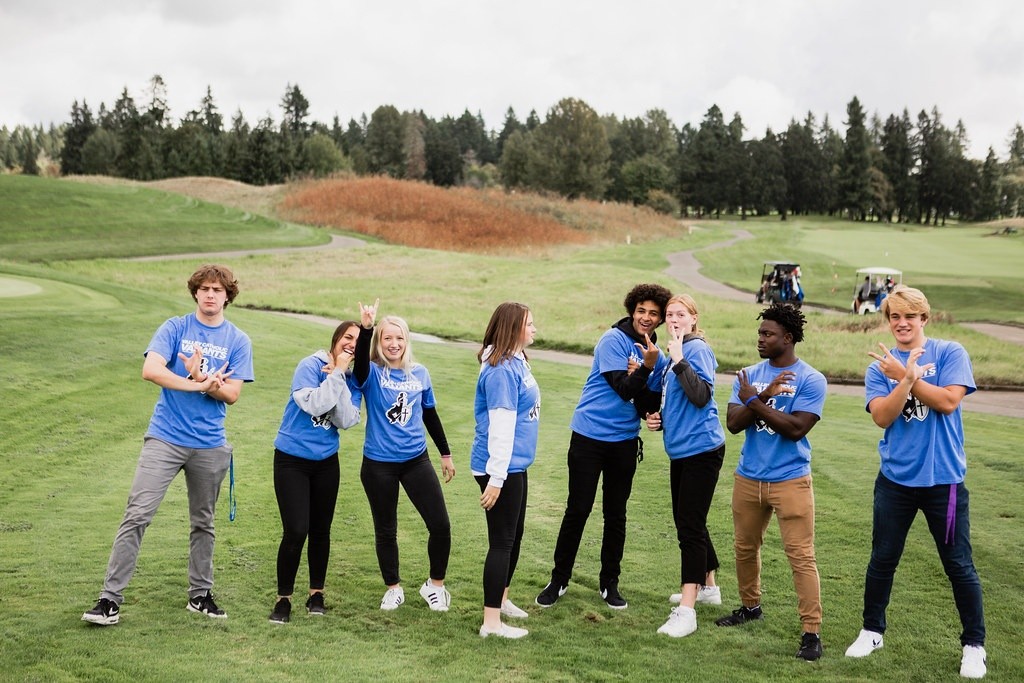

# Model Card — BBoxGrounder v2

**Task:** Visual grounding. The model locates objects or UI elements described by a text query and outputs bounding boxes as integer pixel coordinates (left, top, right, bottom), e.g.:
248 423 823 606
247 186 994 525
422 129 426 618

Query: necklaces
896 347 907 368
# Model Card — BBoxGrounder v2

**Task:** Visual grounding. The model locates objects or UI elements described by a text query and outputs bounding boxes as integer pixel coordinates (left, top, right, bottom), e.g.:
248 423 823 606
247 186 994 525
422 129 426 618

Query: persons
469 302 542 639
857 275 894 303
714 304 828 661
351 298 456 612
80 265 255 626
844 286 987 678
270 320 362 624
535 283 673 608
645 293 725 638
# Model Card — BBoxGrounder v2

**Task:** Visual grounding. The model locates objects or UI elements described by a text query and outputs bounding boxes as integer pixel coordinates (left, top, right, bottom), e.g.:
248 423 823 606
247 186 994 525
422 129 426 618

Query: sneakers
796 631 823 665
669 585 722 605
380 586 405 611
845 629 884 658
479 620 529 640
959 644 988 679
269 597 291 624
714 604 765 626
600 586 627 610
656 605 698 638
500 598 528 618
419 578 451 611
80 598 120 625
186 590 227 618
305 591 325 614
535 581 567 607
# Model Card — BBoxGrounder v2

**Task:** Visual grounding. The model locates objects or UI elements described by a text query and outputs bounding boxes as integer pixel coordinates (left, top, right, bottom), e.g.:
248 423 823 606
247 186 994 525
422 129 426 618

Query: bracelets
201 374 208 382
201 391 206 395
745 395 758 408
440 455 452 458
